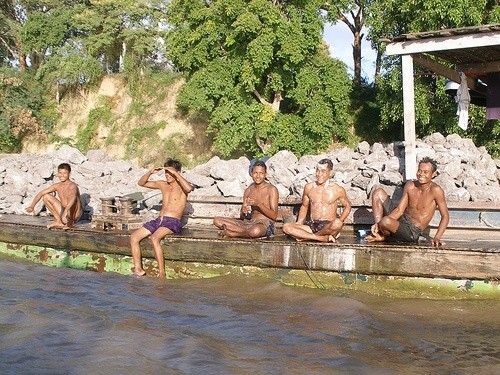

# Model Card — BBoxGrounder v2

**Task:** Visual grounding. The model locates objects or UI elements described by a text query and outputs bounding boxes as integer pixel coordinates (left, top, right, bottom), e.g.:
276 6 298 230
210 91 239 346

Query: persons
363 158 450 246
282 157 351 242
26 163 83 230
129 159 194 276
213 160 278 239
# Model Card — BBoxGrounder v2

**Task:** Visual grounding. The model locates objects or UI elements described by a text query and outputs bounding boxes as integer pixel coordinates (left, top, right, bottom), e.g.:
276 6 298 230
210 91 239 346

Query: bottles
244 197 252 217
